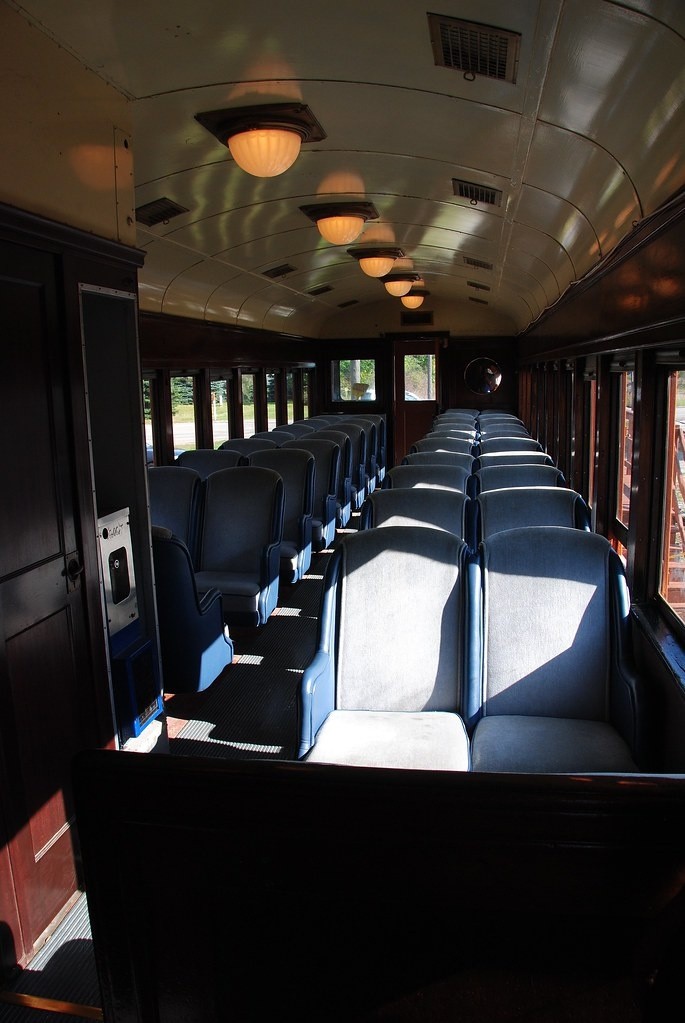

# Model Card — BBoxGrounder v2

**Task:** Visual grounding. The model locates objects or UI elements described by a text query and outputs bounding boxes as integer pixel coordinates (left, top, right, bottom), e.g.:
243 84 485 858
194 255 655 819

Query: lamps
378 275 421 296
196 102 329 177
347 248 405 278
296 201 380 246
400 288 431 309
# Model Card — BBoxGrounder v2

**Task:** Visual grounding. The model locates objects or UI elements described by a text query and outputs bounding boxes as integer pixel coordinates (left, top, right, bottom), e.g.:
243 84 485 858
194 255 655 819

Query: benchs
145 407 644 770
